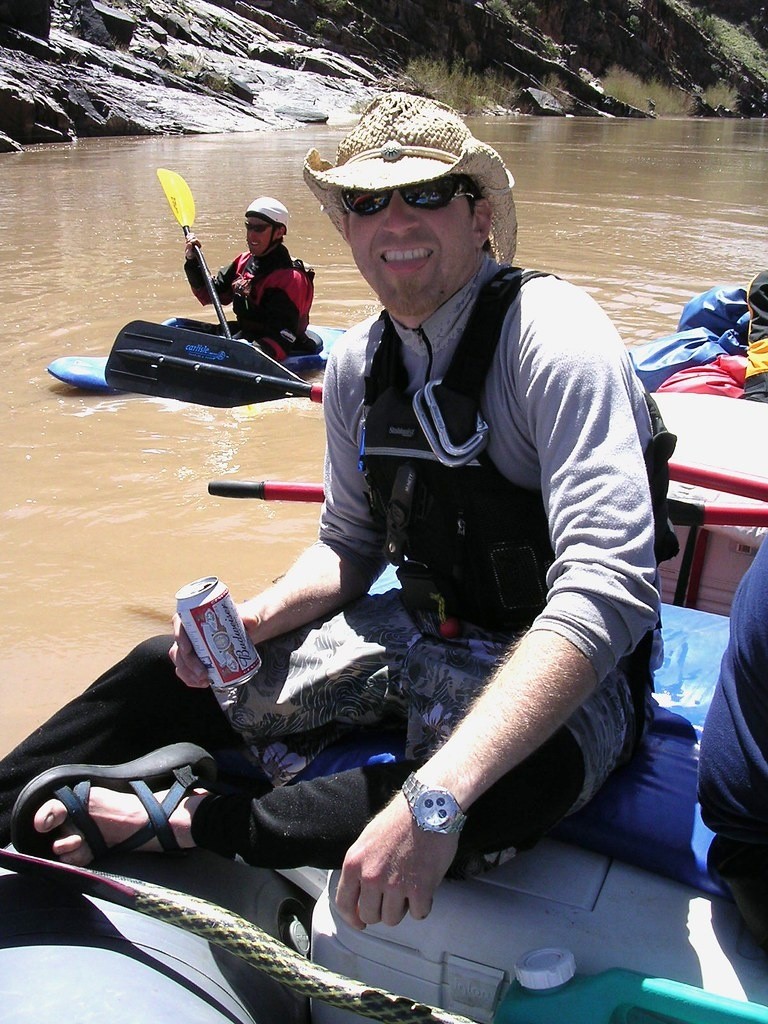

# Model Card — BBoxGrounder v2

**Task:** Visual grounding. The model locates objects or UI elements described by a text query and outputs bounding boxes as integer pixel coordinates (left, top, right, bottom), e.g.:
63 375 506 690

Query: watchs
401 771 467 835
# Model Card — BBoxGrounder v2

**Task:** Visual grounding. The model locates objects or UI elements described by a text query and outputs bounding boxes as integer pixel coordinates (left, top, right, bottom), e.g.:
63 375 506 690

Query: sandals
9 741 219 873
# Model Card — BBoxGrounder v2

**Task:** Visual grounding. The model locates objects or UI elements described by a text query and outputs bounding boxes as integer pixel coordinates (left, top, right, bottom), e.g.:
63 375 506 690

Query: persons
184 196 313 362
0 91 661 932
697 531 768 954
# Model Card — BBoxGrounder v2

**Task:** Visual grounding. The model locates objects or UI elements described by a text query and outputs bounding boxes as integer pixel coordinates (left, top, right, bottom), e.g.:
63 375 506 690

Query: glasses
245 220 271 233
340 174 481 216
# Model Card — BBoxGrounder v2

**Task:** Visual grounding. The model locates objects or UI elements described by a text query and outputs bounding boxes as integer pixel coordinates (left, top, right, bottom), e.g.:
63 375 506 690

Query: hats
244 196 289 236
302 92 519 267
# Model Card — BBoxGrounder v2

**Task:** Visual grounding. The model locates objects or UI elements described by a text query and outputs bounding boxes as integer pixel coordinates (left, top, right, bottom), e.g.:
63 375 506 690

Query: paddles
103 319 768 502
157 167 231 338
209 476 768 529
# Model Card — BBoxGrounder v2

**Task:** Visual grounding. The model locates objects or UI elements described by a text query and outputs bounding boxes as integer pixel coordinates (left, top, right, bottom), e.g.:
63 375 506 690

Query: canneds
175 576 261 689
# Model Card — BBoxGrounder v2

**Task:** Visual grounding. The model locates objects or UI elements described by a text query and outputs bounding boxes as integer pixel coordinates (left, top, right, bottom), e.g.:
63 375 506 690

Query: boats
47 318 348 393
